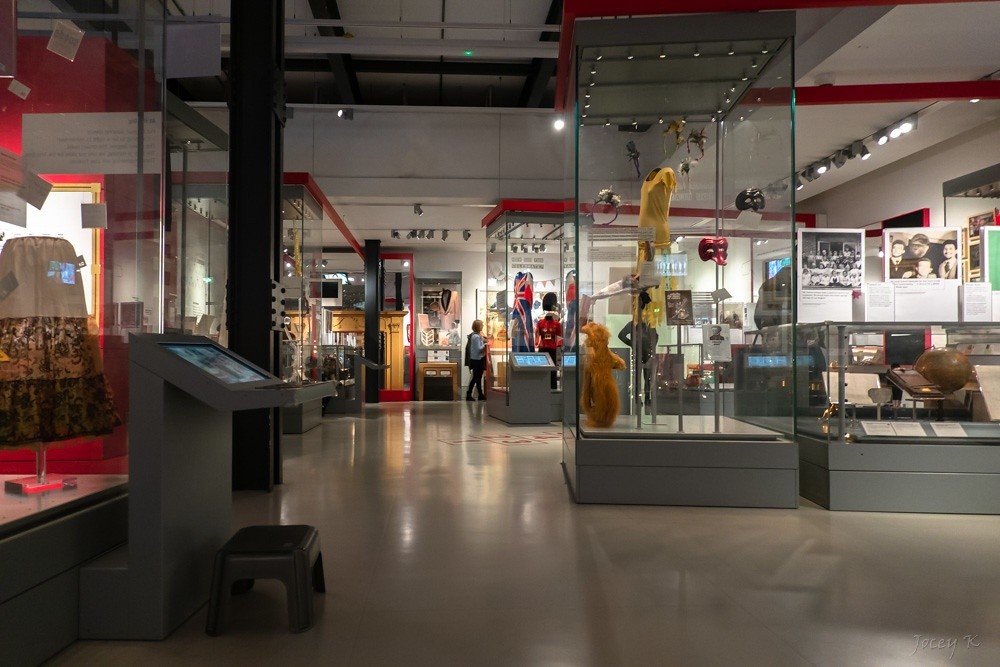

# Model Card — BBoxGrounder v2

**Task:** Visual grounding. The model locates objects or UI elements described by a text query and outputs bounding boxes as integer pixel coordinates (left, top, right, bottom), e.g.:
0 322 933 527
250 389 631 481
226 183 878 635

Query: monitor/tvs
764 257 790 281
305 279 343 307
156 342 271 385
509 351 555 367
561 353 576 367
748 354 816 367
47 260 77 285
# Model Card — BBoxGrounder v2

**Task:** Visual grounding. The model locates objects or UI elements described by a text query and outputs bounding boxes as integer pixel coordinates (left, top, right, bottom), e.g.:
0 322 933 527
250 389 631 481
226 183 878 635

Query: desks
418 362 457 400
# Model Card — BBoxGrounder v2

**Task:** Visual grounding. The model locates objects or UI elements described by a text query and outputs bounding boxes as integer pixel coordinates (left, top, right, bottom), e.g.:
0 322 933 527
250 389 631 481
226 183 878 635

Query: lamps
338 109 354 120
391 229 471 242
793 114 919 190
413 204 424 217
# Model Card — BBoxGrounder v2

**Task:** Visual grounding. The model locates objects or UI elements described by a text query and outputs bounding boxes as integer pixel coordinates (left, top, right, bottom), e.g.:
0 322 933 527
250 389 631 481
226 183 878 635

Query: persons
511 272 536 352
753 264 832 406
619 320 658 406
535 315 562 393
802 244 862 289
631 168 676 327
710 326 724 340
466 320 488 402
889 233 958 280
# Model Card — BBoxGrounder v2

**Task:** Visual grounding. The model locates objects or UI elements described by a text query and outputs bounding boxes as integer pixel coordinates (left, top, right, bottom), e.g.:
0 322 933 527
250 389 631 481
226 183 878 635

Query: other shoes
645 399 651 405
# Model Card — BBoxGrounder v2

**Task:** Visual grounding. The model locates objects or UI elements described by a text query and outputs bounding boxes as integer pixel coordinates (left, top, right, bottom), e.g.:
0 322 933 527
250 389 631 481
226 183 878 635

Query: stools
205 524 326 637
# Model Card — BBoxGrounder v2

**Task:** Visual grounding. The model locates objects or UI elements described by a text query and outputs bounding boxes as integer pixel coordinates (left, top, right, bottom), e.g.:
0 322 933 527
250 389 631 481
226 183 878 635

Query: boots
466 391 476 401
477 390 487 400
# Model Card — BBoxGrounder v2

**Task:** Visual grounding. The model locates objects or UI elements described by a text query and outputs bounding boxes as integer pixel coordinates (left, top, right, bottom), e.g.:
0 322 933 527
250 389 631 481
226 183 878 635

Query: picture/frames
881 211 996 280
